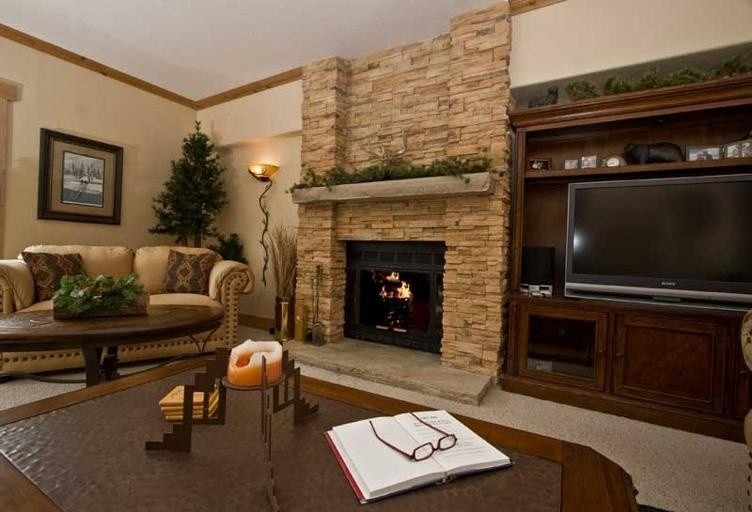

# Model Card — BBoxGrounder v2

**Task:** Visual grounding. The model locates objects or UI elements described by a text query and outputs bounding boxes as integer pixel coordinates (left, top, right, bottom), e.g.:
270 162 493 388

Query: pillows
21 251 88 302
161 249 218 296
133 245 223 294
17 245 134 277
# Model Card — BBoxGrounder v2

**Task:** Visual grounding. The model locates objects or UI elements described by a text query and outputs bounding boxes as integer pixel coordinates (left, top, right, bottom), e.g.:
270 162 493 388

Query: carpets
1 325 749 511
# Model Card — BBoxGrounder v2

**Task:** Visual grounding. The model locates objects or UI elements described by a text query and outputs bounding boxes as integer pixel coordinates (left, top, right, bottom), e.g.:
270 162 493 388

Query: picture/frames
527 158 552 172
578 153 599 168
563 158 579 170
686 144 722 162
724 138 752 159
38 128 124 225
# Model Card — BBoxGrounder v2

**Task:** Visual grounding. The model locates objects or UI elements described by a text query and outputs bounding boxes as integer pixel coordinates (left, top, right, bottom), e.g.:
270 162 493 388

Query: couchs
0 259 256 377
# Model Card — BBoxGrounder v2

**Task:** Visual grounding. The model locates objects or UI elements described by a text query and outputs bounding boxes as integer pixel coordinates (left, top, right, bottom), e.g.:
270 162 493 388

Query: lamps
248 154 280 182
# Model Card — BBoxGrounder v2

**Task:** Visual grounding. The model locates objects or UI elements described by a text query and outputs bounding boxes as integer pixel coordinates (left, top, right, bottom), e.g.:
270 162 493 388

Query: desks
0 353 640 512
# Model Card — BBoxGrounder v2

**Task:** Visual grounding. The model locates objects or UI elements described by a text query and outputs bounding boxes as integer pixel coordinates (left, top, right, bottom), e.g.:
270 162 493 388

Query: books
322 409 516 505
158 382 222 421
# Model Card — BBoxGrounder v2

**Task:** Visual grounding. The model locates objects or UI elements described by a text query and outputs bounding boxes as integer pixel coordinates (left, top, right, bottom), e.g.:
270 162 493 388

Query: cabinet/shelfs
500 74 752 443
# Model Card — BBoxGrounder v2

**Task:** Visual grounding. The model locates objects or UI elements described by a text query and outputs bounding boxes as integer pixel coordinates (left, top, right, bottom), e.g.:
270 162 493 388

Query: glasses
368 409 459 463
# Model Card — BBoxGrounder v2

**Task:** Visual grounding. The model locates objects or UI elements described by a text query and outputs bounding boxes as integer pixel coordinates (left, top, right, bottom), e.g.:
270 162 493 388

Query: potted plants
260 220 296 342
53 271 150 319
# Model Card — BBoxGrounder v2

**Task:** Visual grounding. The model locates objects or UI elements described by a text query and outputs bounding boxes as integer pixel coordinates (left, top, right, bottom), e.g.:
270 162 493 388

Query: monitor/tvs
564 173 752 311
